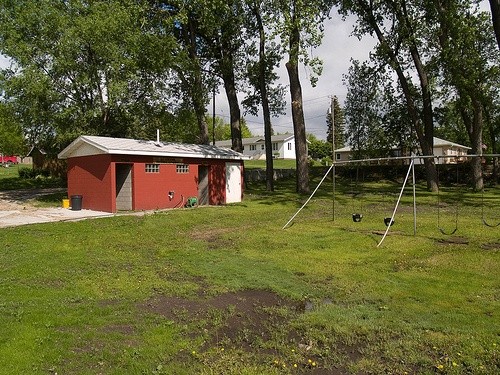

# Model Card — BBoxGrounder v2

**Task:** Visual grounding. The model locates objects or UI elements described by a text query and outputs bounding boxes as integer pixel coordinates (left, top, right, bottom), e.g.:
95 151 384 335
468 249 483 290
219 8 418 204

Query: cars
0 149 21 166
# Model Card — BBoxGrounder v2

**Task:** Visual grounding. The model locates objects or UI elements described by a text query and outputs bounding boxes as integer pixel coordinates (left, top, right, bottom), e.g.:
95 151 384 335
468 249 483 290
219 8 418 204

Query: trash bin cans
61 196 70 209
70 195 82 210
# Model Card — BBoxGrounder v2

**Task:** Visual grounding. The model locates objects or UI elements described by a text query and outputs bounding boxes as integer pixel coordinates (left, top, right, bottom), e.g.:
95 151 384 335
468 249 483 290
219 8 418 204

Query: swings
348 159 366 222
379 156 398 226
436 155 459 236
479 153 500 228
